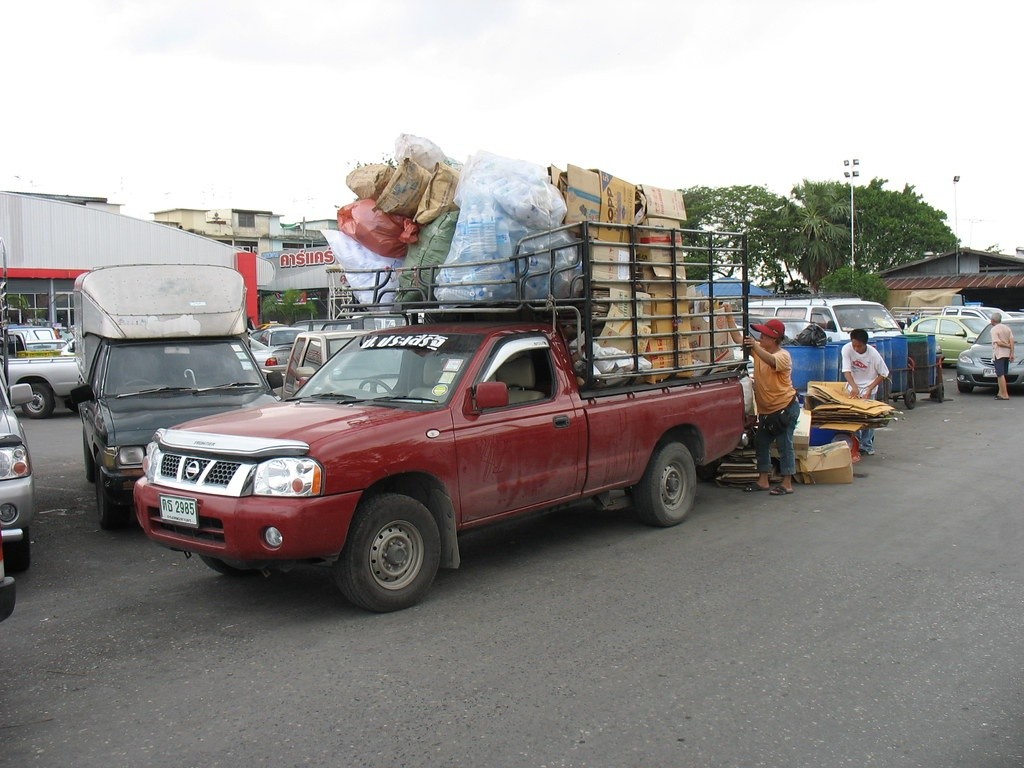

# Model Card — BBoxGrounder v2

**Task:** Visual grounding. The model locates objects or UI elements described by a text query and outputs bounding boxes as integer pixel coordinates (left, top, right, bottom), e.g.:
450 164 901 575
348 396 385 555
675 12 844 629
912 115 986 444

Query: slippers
744 483 769 490
769 484 794 495
995 397 1009 400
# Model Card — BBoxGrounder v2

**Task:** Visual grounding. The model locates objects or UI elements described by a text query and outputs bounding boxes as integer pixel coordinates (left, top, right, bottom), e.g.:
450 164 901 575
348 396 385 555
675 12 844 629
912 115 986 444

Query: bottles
430 153 584 308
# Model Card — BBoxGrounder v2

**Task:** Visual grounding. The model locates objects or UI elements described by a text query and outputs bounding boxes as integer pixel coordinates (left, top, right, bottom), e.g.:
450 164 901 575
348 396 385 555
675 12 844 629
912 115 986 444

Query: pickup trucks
133 221 753 613
4 355 79 420
72 264 283 529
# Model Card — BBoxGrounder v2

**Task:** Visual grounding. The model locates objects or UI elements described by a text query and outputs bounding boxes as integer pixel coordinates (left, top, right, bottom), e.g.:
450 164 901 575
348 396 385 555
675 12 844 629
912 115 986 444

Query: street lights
844 159 859 281
953 175 961 275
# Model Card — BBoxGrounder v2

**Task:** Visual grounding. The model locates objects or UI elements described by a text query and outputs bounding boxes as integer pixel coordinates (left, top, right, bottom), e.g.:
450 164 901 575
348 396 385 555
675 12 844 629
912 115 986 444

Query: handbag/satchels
765 408 790 435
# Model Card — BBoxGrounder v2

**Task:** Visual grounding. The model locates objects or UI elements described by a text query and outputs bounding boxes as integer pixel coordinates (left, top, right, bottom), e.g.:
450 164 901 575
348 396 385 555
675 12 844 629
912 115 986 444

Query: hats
749 319 785 337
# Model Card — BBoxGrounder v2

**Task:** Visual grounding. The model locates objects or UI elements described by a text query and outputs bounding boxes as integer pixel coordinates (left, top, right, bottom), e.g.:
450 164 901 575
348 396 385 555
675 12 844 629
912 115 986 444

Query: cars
247 313 425 400
956 320 1024 393
0 372 36 570
731 292 1023 378
0 324 74 355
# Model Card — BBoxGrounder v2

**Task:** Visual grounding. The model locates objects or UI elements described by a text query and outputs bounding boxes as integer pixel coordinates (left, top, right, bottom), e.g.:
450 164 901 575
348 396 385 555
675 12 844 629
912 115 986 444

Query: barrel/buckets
782 331 937 446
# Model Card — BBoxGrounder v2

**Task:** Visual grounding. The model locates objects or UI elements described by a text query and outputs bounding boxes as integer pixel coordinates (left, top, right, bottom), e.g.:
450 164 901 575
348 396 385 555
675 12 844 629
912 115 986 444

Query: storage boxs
545 159 737 386
713 378 897 486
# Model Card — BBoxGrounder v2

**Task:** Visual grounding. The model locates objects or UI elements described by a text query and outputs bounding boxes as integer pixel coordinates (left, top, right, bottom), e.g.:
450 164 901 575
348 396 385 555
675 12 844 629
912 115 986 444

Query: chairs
407 354 455 405
472 356 546 411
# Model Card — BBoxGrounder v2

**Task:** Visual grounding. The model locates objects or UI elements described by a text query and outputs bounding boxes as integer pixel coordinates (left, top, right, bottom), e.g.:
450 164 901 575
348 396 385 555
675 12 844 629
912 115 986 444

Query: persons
990 313 1015 400
722 302 800 496
841 329 890 455
